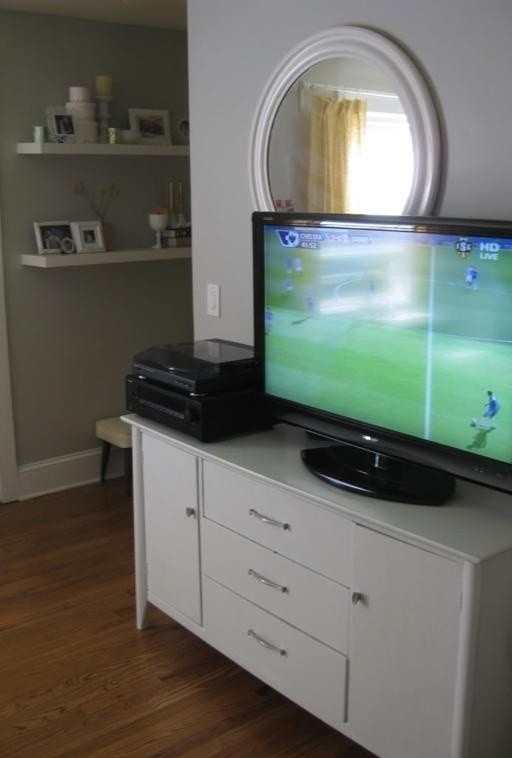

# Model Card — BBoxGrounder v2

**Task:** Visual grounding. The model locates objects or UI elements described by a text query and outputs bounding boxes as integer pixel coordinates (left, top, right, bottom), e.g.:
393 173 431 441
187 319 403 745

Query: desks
119 412 510 758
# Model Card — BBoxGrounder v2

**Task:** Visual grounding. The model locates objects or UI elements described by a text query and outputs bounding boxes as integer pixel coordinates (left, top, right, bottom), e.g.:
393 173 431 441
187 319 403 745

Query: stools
97 419 133 489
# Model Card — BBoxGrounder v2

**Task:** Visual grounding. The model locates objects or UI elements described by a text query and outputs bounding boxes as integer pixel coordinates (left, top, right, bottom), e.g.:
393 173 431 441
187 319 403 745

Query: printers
125 338 273 444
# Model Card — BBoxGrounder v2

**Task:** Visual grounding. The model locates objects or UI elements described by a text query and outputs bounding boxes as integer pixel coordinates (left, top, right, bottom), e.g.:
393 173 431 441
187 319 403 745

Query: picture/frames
126 108 172 144
71 220 106 254
34 221 70 253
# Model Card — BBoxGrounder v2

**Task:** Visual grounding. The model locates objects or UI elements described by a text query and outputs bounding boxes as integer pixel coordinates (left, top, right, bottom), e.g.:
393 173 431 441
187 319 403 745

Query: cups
107 126 121 145
33 125 45 142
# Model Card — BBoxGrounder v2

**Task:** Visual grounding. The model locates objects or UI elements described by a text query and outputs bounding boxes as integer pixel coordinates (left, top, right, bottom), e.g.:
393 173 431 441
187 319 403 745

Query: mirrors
250 26 446 215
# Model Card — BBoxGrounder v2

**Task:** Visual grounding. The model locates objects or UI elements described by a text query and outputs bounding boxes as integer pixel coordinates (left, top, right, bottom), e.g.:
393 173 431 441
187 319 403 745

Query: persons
465 266 473 287
470 267 478 291
283 253 376 319
478 390 499 430
42 229 62 250
265 305 272 336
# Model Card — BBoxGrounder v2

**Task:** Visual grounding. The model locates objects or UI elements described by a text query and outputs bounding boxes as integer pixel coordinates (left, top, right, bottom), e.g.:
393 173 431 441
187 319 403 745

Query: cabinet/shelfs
15 140 191 269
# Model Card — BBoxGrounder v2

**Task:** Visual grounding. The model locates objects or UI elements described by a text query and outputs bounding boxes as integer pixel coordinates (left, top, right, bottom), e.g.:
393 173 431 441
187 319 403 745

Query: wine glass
149 214 166 249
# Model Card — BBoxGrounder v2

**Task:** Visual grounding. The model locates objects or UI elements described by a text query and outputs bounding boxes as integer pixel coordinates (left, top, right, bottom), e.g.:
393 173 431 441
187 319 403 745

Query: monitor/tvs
252 212 512 506
